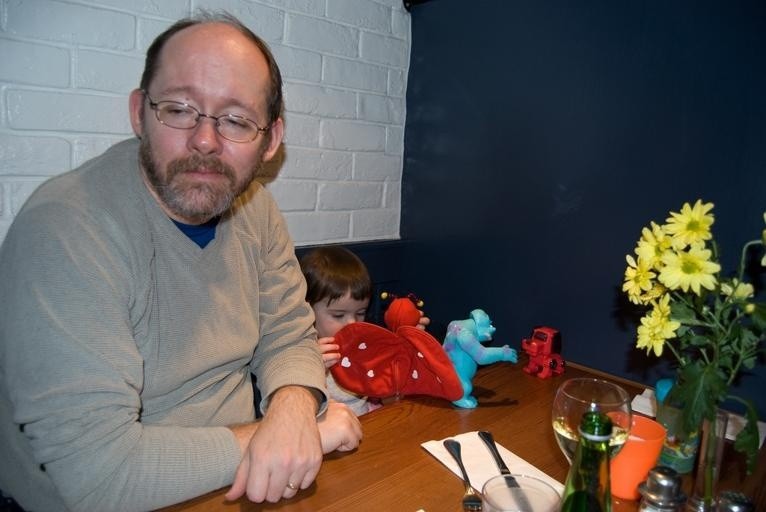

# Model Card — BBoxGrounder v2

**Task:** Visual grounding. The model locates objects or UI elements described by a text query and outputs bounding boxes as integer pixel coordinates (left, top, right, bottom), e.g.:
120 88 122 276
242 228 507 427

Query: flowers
618 191 765 501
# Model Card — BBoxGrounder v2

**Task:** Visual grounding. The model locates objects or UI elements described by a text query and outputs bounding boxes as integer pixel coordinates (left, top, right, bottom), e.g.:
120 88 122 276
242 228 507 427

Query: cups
548 379 634 469
603 411 668 502
480 474 564 510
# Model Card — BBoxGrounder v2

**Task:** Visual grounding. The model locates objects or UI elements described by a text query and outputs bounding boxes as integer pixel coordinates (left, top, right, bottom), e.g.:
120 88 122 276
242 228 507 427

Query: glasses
144 91 274 143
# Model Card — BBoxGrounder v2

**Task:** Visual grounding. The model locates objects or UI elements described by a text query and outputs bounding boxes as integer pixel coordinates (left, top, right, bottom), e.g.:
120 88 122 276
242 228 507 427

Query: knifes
477 428 531 511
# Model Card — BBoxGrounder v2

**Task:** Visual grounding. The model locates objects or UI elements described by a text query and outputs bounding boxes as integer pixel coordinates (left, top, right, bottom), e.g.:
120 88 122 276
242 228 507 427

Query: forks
443 437 483 512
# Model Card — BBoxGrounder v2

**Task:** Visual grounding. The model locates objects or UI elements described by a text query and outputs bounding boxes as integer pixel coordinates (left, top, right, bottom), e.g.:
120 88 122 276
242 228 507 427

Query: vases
686 410 732 511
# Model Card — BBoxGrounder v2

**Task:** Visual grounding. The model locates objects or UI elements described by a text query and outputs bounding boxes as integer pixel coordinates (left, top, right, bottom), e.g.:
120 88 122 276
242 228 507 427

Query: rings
287 481 302 494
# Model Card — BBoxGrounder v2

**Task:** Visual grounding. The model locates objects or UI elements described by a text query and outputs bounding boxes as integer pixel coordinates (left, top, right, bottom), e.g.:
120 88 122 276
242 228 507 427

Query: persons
0 6 366 512
301 243 429 418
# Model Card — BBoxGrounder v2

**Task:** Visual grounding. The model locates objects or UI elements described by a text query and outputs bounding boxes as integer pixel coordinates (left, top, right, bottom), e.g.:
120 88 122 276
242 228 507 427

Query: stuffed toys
328 292 462 403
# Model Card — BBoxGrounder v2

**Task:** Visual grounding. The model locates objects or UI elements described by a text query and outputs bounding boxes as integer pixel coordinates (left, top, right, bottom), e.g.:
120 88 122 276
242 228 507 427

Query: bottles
559 412 613 510
718 488 754 511
636 464 689 512
